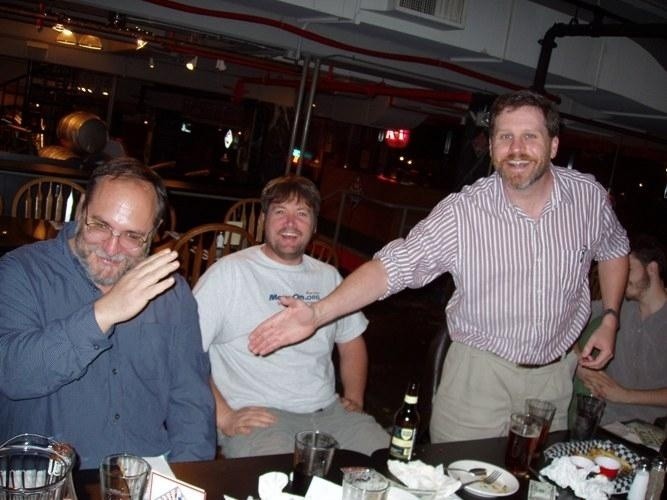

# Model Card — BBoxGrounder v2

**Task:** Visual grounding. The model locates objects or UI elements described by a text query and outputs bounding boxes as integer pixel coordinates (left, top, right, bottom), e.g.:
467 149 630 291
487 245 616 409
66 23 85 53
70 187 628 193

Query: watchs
600 307 623 330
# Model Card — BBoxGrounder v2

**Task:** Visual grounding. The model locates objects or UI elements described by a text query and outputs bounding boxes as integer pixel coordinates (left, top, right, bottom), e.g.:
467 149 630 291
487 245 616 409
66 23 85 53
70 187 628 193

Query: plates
447 457 520 498
542 440 646 496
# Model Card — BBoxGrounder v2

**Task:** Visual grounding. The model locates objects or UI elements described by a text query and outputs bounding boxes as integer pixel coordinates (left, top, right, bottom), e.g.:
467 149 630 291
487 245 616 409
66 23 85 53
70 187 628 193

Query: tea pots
0 433 77 500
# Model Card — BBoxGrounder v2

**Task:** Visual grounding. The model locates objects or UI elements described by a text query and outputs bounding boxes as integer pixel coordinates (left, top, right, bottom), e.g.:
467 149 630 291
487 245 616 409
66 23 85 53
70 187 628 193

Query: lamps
47 20 199 72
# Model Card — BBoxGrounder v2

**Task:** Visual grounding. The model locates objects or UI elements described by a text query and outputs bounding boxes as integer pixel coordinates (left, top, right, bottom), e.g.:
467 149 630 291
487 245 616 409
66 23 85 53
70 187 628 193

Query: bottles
216 231 225 256
626 460 667 500
387 381 423 462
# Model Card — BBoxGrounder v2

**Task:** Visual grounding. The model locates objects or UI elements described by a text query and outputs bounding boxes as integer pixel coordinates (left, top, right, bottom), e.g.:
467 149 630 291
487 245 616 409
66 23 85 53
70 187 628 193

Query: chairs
158 194 340 292
9 176 85 224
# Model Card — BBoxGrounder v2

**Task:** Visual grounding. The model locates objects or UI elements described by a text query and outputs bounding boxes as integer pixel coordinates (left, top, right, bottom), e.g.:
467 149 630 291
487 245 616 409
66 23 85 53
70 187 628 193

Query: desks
330 186 432 261
0 214 60 257
73 418 664 500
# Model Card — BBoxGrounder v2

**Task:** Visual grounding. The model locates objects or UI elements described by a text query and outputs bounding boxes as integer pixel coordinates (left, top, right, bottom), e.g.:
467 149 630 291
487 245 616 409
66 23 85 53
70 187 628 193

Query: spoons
444 467 486 477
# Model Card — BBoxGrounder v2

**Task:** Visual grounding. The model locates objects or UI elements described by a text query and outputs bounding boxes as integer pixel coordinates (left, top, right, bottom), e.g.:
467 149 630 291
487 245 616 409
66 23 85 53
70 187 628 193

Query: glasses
85 205 159 248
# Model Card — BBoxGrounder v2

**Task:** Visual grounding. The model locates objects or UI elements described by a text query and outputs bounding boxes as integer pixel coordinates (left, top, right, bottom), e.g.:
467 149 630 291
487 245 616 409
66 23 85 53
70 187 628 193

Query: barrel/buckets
56 110 108 155
38 144 83 164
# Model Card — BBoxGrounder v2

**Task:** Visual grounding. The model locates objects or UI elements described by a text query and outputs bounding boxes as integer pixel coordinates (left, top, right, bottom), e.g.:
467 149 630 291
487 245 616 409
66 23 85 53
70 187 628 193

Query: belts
520 346 573 368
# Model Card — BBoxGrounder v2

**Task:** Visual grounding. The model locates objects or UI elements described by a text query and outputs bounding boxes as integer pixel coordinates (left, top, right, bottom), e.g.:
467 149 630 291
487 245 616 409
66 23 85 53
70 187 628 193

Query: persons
246 89 633 447
189 170 394 459
575 233 667 430
1 153 216 483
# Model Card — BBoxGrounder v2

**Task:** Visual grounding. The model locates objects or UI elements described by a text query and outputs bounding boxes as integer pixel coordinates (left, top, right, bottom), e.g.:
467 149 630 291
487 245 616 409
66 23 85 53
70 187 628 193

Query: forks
451 469 501 495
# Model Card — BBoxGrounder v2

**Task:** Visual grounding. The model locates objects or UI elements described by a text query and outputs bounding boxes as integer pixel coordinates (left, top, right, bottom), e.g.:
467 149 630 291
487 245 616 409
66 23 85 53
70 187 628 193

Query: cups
564 383 606 441
596 455 621 480
98 451 152 500
289 428 339 490
570 455 596 470
341 465 392 499
503 397 558 475
